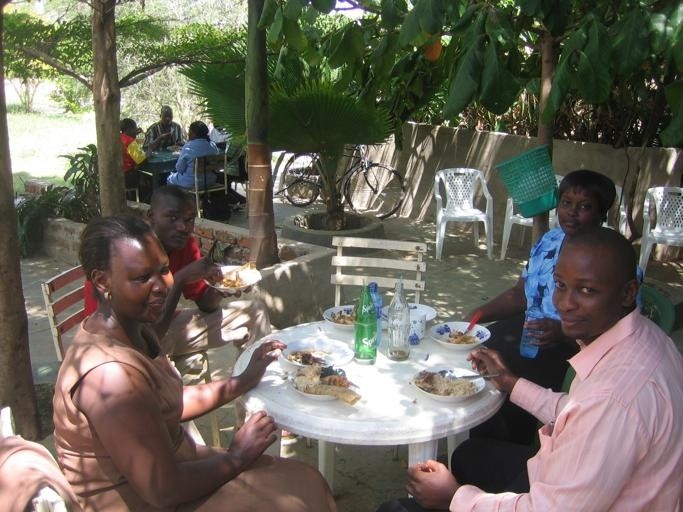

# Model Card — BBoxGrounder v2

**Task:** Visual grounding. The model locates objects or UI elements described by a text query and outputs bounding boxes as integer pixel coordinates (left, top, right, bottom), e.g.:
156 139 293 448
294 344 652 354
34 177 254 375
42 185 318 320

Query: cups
408 309 426 344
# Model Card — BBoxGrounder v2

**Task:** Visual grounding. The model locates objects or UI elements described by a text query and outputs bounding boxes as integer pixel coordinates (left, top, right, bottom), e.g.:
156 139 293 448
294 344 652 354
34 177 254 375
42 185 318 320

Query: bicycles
273 141 405 219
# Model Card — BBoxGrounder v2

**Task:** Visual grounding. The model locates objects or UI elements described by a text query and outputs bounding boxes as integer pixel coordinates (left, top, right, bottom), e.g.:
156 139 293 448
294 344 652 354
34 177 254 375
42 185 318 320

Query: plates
383 303 437 324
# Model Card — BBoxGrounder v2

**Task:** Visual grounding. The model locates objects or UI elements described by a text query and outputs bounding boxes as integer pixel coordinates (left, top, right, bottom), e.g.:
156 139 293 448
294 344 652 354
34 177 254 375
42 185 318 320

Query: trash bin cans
493 143 560 218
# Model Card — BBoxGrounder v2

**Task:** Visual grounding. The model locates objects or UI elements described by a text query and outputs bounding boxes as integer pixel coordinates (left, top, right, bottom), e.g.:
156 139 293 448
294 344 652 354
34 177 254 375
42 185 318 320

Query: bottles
367 283 382 346
520 297 544 358
387 282 410 361
354 285 376 363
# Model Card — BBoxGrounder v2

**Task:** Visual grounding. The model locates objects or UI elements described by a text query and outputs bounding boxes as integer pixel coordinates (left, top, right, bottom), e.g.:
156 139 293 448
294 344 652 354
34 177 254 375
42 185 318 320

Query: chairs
638 184 683 274
500 171 564 262
601 186 627 238
433 168 495 262
127 142 246 218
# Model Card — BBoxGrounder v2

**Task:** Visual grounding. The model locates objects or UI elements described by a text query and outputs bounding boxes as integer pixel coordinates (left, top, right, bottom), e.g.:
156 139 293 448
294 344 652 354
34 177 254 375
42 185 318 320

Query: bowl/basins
205 266 262 294
429 321 492 350
279 337 355 368
412 366 484 403
323 305 353 329
292 366 376 400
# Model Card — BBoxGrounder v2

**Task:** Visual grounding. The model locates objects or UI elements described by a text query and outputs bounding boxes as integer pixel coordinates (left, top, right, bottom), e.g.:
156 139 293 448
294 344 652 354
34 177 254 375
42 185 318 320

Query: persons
462 168 645 441
207 119 249 205
142 105 185 151
167 120 221 189
82 183 270 446
375 225 682 512
51 211 339 511
119 118 154 206
